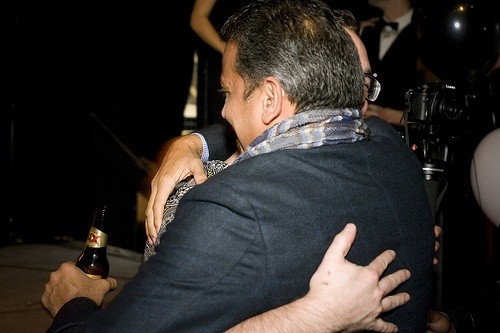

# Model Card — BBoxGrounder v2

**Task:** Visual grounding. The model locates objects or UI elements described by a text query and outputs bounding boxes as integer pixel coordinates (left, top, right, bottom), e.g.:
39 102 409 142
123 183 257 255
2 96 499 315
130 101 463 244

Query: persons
41 0 442 333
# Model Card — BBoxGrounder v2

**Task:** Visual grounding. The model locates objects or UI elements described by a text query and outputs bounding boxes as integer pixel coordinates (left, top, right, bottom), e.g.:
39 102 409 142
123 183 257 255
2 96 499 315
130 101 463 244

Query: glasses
362 75 381 101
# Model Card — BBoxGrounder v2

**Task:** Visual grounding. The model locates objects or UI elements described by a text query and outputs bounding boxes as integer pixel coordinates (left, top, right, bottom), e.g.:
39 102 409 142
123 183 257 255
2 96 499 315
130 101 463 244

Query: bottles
76 206 113 279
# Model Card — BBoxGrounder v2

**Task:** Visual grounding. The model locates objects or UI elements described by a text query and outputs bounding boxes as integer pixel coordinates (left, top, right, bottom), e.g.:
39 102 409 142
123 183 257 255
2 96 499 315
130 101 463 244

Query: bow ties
378 20 398 32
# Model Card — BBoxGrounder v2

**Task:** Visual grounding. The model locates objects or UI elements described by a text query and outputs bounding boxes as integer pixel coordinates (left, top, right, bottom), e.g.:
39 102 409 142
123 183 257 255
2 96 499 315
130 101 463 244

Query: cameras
408 81 476 128
